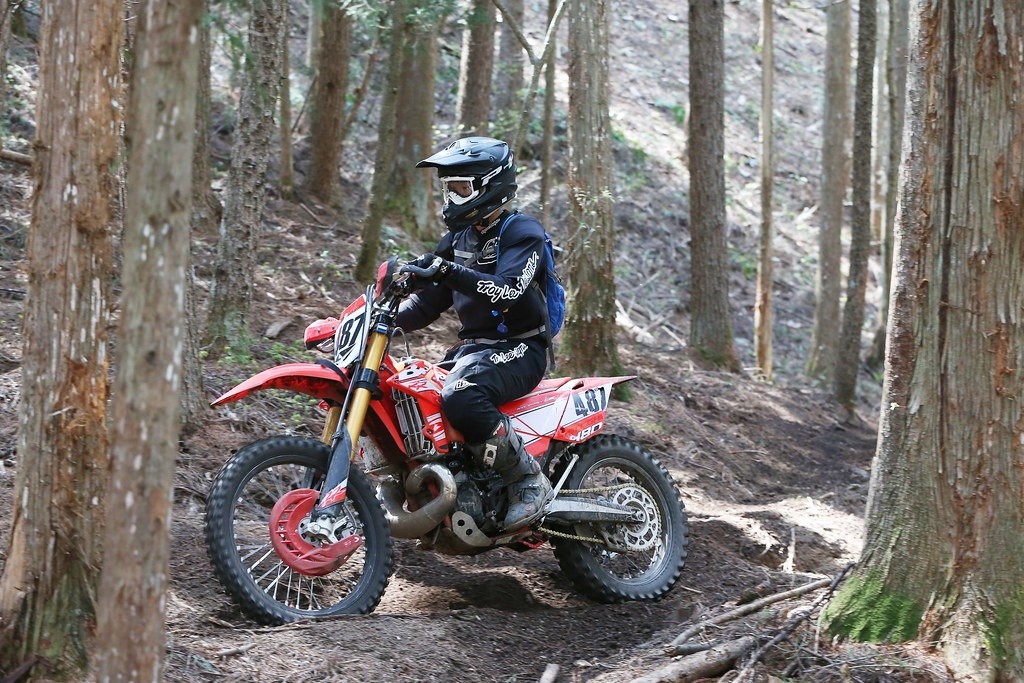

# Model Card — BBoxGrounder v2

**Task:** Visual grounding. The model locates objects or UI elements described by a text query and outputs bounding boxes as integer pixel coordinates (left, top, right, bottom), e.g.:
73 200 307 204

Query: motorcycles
199 258 693 621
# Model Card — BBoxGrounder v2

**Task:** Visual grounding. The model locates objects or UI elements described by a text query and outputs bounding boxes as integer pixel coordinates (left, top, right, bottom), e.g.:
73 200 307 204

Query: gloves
400 253 451 291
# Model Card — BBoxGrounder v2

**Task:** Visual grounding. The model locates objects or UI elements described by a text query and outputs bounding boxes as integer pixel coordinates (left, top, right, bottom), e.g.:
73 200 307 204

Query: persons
373 137 553 527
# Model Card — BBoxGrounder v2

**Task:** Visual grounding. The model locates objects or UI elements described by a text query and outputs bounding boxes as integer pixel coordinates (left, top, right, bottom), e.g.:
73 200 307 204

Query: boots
465 411 554 533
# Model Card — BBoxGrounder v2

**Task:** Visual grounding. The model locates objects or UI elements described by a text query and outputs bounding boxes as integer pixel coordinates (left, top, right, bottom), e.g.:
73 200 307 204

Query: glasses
440 176 477 206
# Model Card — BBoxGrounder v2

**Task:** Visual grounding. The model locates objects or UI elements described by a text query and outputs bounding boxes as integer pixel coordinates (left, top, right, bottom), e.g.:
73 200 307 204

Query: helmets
415 136 518 233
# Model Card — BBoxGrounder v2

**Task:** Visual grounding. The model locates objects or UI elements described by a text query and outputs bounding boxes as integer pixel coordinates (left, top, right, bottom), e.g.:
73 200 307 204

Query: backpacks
492 214 565 339
